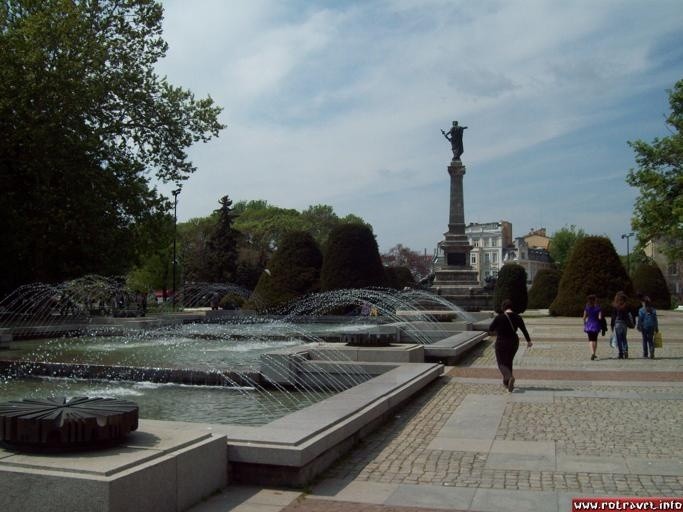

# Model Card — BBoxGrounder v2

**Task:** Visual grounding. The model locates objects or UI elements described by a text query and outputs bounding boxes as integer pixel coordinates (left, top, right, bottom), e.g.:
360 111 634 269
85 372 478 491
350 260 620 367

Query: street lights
621 232 634 271
171 188 181 309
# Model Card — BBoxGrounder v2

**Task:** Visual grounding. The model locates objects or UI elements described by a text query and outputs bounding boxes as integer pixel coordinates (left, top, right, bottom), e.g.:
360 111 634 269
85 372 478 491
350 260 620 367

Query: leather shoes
590 350 655 360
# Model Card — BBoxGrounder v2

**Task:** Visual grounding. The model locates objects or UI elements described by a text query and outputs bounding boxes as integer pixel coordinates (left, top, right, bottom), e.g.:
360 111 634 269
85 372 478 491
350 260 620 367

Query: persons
637 296 659 358
446 121 468 158
609 291 636 359
583 295 602 361
489 298 533 393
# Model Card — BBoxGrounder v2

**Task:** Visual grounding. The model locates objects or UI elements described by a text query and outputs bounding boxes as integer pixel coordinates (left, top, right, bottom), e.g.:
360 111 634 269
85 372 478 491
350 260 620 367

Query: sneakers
507 377 513 392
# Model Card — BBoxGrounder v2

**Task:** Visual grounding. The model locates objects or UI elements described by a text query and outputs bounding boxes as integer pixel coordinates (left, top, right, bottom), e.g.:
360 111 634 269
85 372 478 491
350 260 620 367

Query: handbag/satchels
653 333 662 348
609 333 617 349
